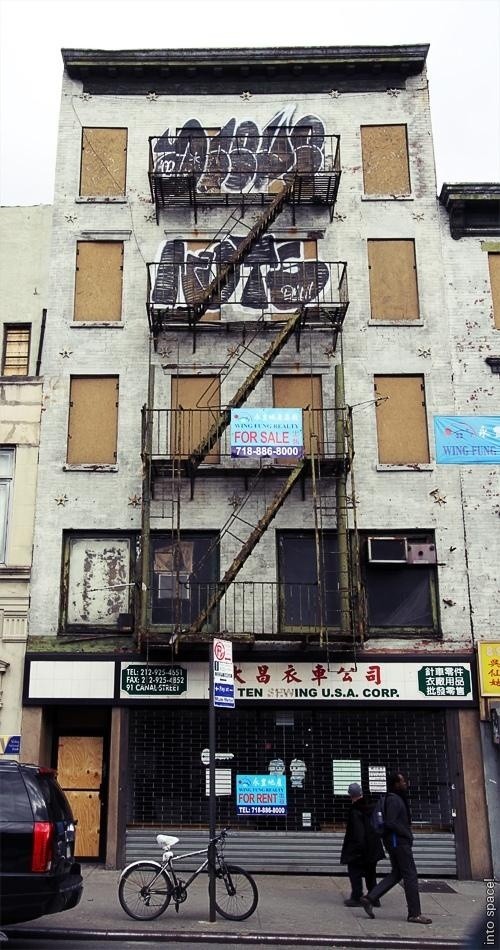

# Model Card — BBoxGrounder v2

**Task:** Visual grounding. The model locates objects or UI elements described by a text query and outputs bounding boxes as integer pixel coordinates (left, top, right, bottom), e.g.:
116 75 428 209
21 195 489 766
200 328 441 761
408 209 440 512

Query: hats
348 783 360 796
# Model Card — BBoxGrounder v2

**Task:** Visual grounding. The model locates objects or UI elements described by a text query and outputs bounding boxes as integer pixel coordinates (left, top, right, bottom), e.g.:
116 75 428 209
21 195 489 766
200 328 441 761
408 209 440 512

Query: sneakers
343 897 363 906
407 915 433 924
360 896 380 919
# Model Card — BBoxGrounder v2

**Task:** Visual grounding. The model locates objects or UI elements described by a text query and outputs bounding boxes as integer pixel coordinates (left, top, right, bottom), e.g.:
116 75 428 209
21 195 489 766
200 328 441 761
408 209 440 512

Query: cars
0 758 85 927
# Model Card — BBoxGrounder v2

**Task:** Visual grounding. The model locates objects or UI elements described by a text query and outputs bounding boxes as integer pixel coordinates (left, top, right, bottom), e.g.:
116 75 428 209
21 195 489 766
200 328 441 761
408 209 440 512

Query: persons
340 782 386 908
359 772 433 924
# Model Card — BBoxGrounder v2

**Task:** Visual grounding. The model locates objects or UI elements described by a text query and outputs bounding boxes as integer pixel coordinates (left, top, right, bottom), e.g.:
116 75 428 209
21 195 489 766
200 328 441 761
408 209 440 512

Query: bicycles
117 826 259 922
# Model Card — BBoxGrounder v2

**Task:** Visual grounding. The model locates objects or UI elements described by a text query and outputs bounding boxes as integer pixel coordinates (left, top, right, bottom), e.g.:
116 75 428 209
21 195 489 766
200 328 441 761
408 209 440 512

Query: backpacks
372 792 402 836
